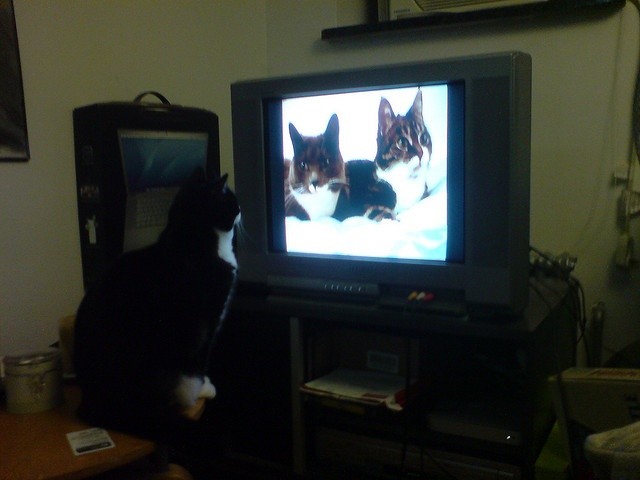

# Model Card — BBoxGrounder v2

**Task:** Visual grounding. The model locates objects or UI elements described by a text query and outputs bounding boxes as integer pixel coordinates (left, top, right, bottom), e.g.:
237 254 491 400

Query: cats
283 114 363 223
72 165 254 447
345 93 434 222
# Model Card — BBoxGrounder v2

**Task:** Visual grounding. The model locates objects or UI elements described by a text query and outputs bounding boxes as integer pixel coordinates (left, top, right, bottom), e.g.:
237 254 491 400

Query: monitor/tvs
229 53 533 329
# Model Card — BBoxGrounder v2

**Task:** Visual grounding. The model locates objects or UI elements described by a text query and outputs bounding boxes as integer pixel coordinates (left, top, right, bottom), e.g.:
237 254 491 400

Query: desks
2 406 156 479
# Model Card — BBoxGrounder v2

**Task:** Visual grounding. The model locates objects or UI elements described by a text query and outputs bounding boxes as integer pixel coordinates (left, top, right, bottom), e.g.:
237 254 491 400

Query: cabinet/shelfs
246 277 580 480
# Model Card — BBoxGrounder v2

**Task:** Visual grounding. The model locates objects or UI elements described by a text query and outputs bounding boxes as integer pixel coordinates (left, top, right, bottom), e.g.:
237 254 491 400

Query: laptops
118 129 211 258
